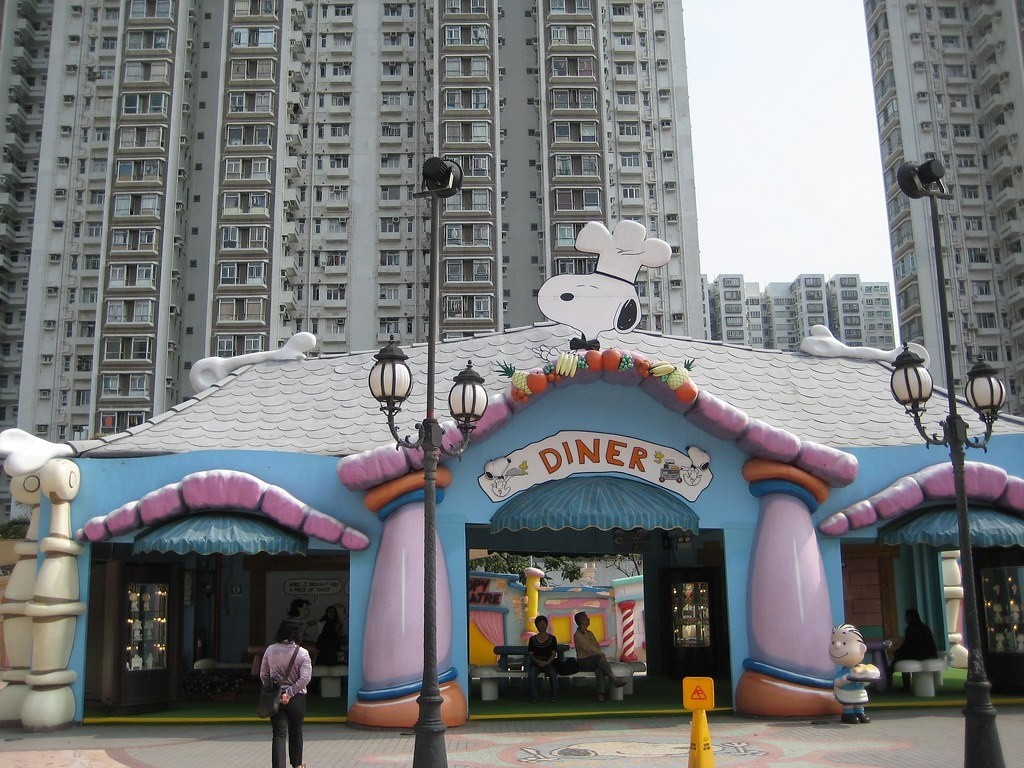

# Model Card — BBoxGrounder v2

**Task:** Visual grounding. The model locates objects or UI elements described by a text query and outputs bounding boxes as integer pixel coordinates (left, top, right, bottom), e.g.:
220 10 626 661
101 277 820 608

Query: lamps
125 584 167 653
983 576 1019 632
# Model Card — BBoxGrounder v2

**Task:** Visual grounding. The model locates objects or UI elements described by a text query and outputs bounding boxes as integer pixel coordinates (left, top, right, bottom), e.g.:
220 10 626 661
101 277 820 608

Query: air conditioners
968 320 977 330
41 0 645 432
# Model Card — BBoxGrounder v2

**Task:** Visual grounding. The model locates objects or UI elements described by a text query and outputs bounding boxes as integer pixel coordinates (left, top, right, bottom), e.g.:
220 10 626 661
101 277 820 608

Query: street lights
368 155 489 767
890 158 1006 768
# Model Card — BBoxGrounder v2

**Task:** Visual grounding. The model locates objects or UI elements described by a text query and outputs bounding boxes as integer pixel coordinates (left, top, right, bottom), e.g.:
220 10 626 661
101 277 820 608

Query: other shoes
550 690 560 698
531 698 536 702
294 764 306 768
615 680 628 688
598 695 604 702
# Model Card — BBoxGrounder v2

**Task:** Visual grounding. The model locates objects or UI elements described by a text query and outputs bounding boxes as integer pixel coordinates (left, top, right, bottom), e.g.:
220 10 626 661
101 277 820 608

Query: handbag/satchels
256 687 283 718
558 658 579 675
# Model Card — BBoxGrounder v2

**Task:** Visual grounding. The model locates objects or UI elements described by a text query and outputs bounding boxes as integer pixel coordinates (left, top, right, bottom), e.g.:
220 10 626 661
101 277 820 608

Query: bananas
555 352 579 378
650 360 677 377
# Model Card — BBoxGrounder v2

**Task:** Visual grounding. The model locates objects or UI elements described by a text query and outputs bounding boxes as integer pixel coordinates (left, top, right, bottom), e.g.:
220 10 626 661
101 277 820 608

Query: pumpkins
601 347 622 371
674 379 696 403
586 350 602 371
527 371 547 393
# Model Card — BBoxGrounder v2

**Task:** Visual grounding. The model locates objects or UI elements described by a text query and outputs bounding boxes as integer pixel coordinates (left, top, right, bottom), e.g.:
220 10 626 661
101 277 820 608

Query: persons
309 604 343 694
259 619 312 768
573 611 628 702
525 616 561 703
886 609 936 691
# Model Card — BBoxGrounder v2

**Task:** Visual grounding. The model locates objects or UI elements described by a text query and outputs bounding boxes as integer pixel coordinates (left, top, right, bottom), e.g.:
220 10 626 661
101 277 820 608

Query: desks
863 639 893 693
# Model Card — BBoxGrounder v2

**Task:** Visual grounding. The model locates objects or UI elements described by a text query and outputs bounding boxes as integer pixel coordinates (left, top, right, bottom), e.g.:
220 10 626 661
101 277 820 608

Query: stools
894 658 947 697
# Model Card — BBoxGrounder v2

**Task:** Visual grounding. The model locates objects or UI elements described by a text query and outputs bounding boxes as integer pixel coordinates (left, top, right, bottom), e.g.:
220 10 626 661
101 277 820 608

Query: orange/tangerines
511 389 528 403
547 371 561 382
634 358 650 377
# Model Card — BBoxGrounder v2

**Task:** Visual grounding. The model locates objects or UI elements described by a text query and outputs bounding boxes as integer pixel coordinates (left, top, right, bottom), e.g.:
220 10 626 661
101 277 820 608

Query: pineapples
490 360 532 394
667 359 696 390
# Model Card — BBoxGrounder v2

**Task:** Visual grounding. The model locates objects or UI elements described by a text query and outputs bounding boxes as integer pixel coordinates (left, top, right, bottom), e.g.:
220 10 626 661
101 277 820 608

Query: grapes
577 355 589 368
619 355 633 372
544 362 555 374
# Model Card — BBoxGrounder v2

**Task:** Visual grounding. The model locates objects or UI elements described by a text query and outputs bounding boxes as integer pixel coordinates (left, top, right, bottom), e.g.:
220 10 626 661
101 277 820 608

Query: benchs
194 659 348 698
468 661 646 702
